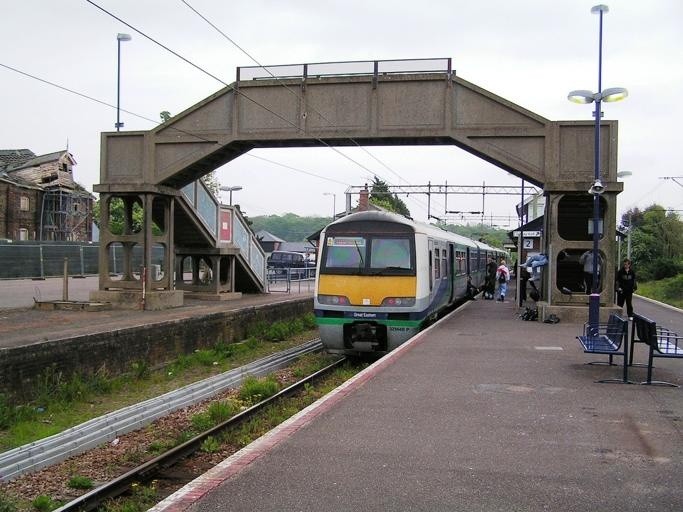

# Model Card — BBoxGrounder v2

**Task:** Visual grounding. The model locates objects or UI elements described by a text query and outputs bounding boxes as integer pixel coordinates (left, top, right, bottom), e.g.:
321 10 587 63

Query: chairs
575 311 681 387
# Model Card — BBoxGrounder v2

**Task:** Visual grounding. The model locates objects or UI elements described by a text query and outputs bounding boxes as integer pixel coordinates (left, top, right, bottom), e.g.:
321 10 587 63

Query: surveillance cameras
592 181 605 194
587 185 595 196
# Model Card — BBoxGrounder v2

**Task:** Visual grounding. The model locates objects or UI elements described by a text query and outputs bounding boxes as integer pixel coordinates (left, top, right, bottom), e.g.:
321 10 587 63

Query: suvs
265 251 315 275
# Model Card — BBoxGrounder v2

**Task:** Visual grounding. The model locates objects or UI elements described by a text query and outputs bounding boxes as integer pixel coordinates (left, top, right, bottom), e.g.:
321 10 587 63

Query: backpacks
499 272 505 283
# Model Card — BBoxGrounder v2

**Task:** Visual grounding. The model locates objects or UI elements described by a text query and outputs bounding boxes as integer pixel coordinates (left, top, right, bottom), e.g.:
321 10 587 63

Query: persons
480 251 566 303
613 257 637 322
578 250 604 296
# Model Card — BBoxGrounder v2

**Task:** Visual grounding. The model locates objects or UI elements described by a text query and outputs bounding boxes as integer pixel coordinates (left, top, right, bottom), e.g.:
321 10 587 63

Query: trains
310 209 512 358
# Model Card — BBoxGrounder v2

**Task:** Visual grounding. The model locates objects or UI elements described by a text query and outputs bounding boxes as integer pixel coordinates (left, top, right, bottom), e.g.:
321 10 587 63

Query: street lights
218 184 242 204
321 191 335 220
111 31 133 131
587 3 609 120
566 88 628 340
